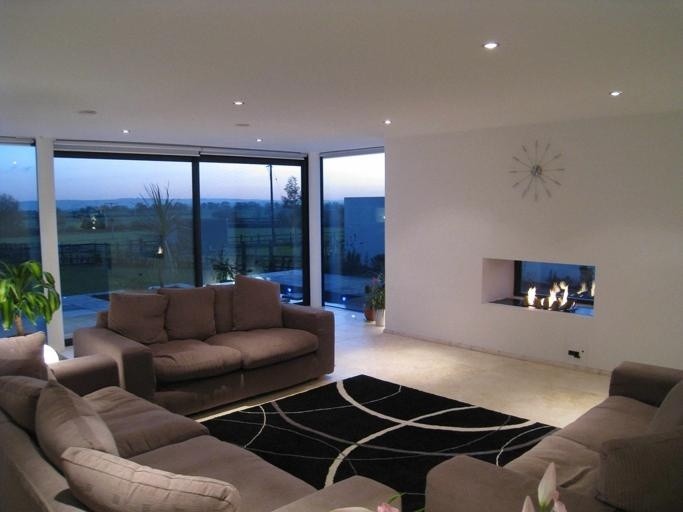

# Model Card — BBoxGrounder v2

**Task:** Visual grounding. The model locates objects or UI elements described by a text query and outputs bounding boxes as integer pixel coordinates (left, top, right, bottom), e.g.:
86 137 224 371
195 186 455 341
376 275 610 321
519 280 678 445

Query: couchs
72 302 335 415
422 359 682 512
1 352 403 511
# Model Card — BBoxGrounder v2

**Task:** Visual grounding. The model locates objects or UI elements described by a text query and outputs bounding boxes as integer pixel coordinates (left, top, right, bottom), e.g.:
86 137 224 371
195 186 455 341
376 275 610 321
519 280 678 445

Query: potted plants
364 285 386 327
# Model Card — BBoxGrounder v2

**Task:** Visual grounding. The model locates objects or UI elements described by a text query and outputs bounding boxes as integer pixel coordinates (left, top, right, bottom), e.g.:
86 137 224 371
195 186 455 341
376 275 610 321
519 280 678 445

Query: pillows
595 379 683 512
1 332 47 380
1 371 44 429
60 444 241 511
108 275 281 343
33 380 118 466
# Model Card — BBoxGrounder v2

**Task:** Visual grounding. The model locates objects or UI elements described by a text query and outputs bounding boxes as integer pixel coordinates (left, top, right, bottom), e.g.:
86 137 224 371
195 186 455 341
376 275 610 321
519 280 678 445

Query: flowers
520 462 568 512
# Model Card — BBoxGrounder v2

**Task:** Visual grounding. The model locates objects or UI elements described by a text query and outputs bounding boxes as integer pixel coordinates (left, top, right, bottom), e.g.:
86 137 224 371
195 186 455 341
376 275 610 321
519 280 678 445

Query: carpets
198 373 563 511
